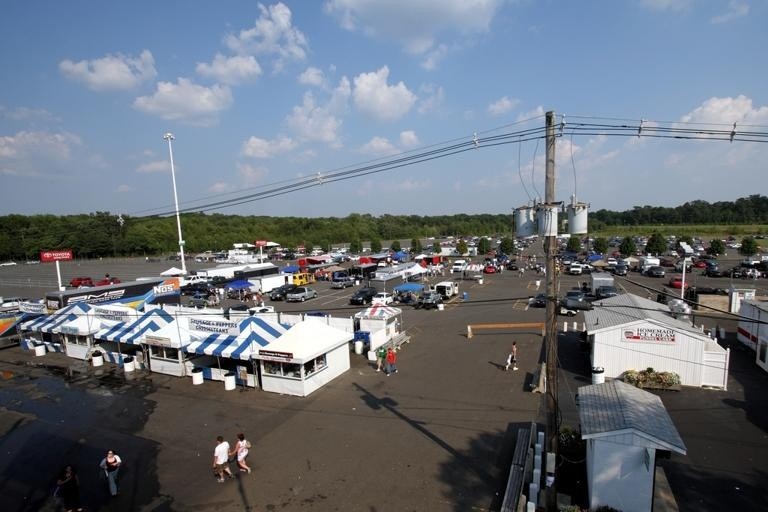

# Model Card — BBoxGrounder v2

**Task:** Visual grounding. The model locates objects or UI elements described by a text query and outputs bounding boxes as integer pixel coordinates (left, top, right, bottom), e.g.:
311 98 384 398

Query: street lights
162 133 188 276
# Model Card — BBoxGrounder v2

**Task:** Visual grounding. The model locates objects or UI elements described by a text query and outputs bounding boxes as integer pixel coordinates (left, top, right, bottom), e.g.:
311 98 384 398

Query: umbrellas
323 265 346 272
224 279 255 299
588 255 602 261
590 259 608 267
621 256 639 262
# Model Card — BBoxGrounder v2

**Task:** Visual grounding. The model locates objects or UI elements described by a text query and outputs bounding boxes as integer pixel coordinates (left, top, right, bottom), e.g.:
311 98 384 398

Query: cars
0 261 18 267
524 232 767 318
146 257 161 263
94 276 120 287
25 260 40 265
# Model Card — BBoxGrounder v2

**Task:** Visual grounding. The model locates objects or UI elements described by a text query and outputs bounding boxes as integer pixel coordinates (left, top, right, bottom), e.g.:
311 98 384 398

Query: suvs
69 276 92 289
0 296 43 311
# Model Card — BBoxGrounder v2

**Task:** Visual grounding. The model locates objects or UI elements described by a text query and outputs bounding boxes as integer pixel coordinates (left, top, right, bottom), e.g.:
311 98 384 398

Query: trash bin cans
92 350 105 366
592 367 604 385
191 368 204 385
463 292 468 300
535 279 542 287
34 344 46 357
354 341 363 356
224 372 236 391
123 357 135 373
479 277 483 285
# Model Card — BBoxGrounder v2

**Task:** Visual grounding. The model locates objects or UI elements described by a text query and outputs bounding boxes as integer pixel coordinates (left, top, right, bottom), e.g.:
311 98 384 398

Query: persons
194 287 265 308
212 435 236 483
375 346 386 372
54 464 83 511
99 448 122 497
228 433 252 473
505 341 519 370
494 252 545 291
384 347 398 377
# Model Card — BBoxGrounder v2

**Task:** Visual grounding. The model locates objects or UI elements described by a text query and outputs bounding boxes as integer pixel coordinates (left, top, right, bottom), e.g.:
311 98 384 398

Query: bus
45 276 180 315
